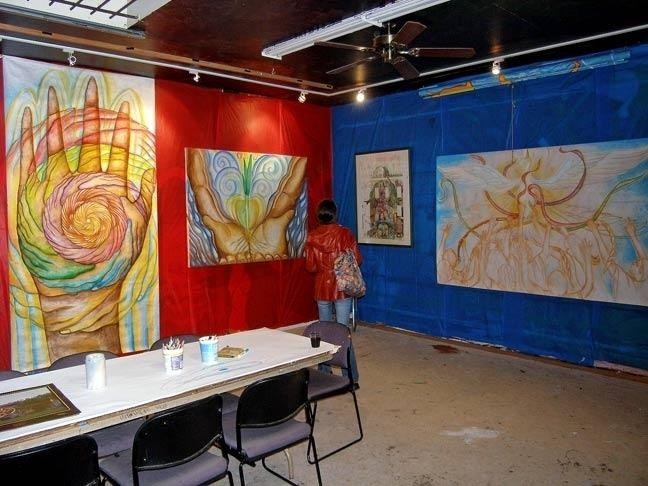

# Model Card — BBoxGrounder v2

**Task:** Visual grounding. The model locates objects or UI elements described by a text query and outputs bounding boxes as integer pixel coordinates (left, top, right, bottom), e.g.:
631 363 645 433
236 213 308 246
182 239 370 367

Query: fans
313 22 477 79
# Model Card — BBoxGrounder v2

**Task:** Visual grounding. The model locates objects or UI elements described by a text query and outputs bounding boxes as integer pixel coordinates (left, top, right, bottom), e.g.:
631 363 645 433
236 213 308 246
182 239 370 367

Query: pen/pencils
237 348 249 358
161 336 185 350
201 334 218 341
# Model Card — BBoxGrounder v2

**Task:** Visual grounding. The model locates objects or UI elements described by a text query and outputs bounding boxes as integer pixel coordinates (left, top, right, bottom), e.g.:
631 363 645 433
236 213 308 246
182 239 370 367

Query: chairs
215 368 322 486
0 434 101 486
301 321 363 465
83 394 234 486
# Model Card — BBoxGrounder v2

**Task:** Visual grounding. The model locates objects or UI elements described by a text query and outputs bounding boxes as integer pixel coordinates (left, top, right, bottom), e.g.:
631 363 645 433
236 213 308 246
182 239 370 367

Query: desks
0 326 340 456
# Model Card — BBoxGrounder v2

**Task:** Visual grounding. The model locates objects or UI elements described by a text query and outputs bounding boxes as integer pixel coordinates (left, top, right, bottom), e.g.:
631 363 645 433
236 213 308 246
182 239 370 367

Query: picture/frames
0 383 82 434
354 147 412 246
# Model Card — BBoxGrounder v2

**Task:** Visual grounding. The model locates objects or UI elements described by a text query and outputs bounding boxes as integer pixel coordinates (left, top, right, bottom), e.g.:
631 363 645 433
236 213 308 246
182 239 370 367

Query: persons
304 200 362 388
17 75 156 366
